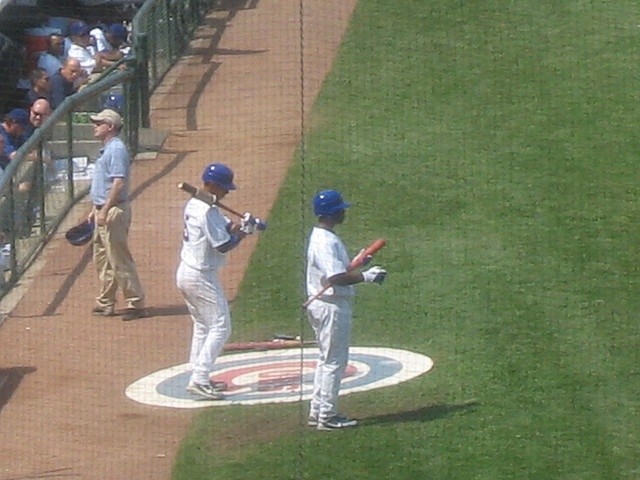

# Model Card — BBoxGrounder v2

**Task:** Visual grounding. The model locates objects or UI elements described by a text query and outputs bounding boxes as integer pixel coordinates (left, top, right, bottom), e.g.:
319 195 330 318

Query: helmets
315 191 350 216
201 164 236 189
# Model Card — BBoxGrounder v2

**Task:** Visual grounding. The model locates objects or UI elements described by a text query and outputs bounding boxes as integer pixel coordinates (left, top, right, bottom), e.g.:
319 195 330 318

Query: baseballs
273 339 284 342
295 336 300 340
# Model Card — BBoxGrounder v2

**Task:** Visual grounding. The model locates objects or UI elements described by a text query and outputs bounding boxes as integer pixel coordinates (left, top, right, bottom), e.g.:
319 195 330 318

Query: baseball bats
178 182 267 230
224 341 317 350
300 239 384 309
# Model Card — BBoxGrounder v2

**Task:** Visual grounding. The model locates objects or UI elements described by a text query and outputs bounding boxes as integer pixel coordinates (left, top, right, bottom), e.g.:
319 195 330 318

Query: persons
303 189 387 432
0 69 63 243
36 19 136 96
174 162 262 399
87 110 146 322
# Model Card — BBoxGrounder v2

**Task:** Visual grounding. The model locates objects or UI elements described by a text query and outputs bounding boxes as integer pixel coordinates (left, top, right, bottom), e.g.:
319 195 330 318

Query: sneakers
92 307 113 315
121 308 146 321
309 413 347 427
210 381 227 391
317 419 357 431
185 380 225 399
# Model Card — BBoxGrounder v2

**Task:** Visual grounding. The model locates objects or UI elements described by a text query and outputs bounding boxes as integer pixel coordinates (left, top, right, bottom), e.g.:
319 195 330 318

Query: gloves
241 217 260 231
362 265 387 284
240 212 260 234
352 248 372 268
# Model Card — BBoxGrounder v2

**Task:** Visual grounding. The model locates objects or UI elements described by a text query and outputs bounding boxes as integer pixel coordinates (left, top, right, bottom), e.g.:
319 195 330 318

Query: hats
70 20 91 35
89 109 124 129
109 23 129 44
5 108 29 121
104 89 125 106
